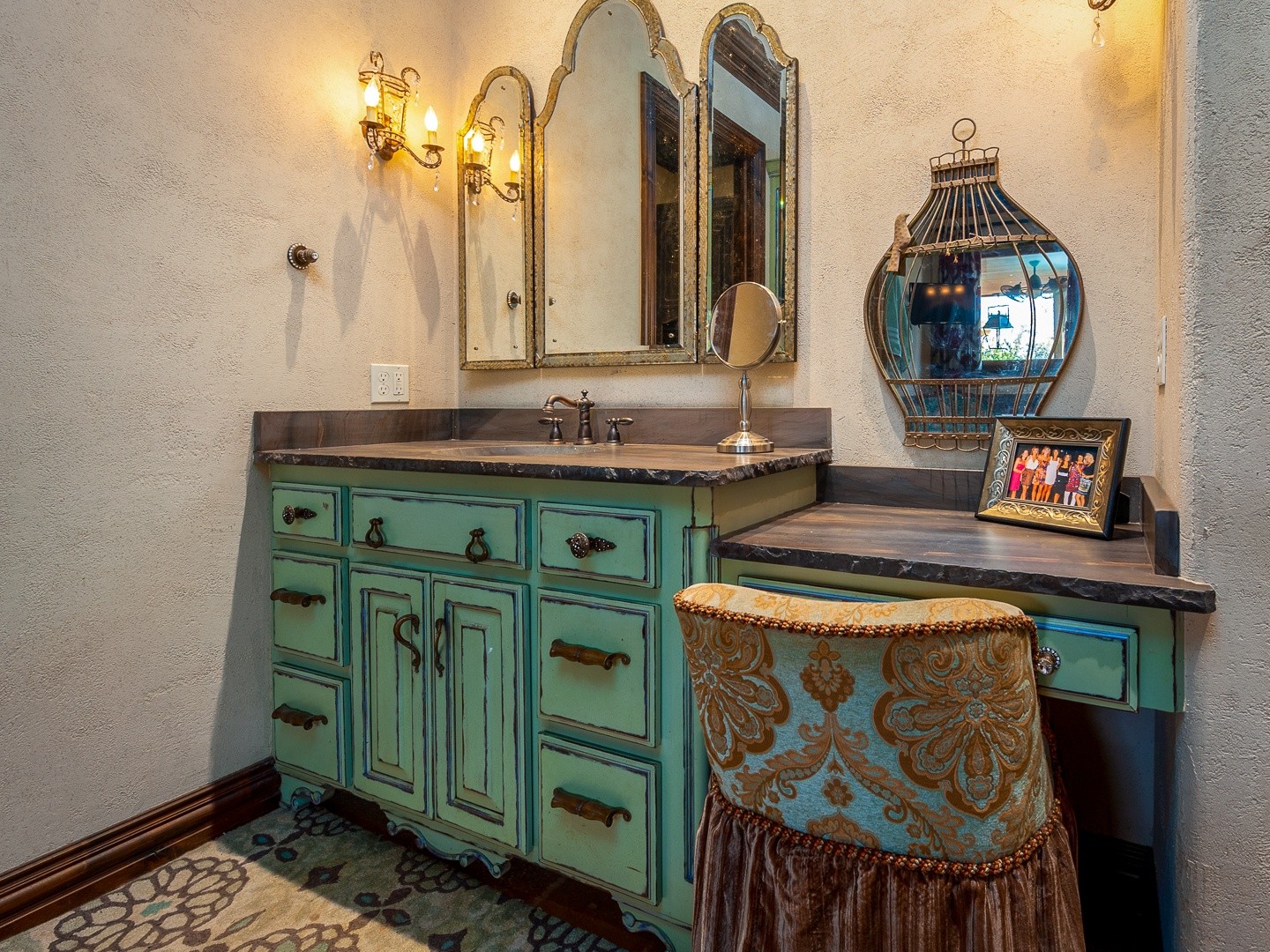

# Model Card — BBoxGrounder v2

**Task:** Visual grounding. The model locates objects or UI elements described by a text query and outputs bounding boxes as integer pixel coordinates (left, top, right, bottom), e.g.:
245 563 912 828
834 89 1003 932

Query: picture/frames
972 415 1135 541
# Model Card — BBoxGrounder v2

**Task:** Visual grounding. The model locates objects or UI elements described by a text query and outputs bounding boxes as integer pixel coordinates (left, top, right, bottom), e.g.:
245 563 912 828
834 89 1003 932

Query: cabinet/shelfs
259 426 825 928
719 499 1189 941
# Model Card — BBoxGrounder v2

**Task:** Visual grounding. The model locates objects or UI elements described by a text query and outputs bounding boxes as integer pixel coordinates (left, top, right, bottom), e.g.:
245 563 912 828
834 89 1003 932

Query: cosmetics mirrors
705 281 787 454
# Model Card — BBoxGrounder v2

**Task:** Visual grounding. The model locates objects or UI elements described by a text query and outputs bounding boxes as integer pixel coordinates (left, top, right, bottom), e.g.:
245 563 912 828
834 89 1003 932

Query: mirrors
453 0 802 370
704 280 789 455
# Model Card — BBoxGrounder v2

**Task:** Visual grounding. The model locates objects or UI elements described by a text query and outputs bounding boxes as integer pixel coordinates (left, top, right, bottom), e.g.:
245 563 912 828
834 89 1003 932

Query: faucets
542 389 598 446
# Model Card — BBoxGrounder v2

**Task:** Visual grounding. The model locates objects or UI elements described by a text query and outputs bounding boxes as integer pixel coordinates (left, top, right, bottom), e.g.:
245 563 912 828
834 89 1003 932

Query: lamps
462 115 521 202
357 50 445 172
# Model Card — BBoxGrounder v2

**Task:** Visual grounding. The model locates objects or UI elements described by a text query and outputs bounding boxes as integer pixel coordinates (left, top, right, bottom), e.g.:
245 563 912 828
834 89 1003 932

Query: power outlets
372 362 410 405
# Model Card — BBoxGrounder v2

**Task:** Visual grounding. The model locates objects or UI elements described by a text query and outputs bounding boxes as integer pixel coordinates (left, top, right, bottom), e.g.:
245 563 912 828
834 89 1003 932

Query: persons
1063 455 1085 505
1041 449 1061 502
1031 446 1051 501
1053 454 1072 503
1009 450 1028 498
1075 453 1095 506
1020 445 1039 499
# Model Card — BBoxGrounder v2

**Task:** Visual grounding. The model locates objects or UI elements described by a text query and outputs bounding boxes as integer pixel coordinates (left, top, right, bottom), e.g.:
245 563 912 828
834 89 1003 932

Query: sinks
431 444 611 460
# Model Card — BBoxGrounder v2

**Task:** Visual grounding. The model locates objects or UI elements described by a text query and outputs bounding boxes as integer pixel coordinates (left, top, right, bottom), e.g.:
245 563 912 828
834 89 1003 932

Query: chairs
676 577 1084 952
